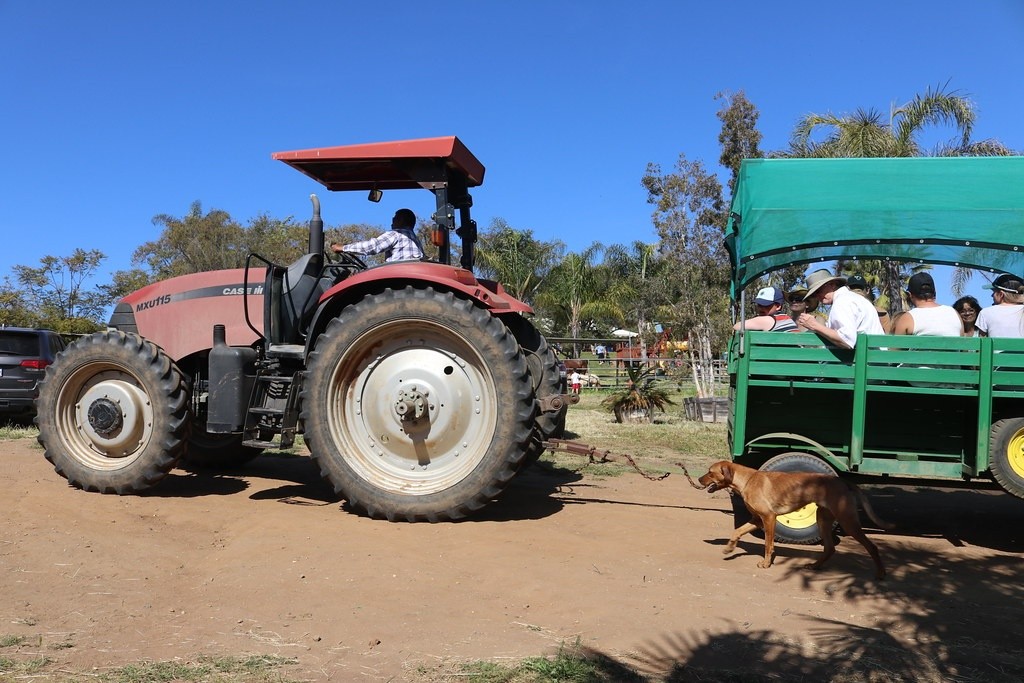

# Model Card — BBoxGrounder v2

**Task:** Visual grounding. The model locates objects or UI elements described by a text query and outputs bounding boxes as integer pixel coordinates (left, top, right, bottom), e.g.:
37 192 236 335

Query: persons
799 268 890 385
332 208 425 262
781 285 825 333
953 295 983 370
974 273 1024 353
595 342 613 365
847 275 890 327
732 288 799 381
570 368 581 395
889 272 964 388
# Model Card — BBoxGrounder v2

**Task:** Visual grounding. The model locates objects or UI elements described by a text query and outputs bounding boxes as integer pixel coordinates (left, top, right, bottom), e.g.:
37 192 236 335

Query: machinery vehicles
33 136 582 523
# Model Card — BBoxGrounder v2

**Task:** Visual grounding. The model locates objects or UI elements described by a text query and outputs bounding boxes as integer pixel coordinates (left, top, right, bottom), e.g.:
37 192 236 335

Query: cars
0 326 68 426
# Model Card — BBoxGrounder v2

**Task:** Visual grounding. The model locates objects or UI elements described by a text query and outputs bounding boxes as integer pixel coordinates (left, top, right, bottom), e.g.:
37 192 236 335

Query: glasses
789 296 803 302
960 308 976 315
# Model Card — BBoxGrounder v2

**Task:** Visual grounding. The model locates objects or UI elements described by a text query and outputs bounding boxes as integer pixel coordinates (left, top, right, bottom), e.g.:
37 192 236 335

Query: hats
906 272 935 293
802 269 847 301
848 276 866 287
782 284 818 312
752 287 784 307
982 273 1024 294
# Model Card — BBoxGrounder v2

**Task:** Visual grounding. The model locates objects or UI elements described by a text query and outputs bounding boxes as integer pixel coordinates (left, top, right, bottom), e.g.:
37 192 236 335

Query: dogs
699 461 896 582
